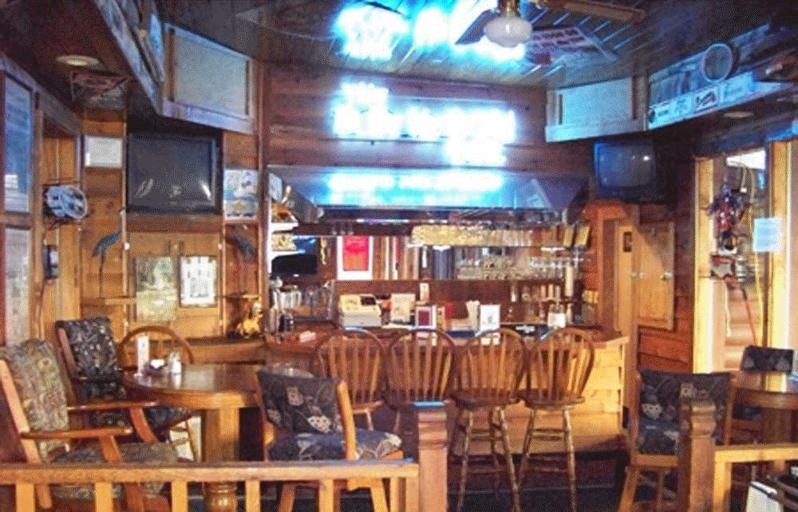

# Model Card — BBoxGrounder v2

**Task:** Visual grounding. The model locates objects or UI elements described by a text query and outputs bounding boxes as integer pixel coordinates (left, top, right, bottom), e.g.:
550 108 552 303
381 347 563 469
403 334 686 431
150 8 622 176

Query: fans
454 0 645 46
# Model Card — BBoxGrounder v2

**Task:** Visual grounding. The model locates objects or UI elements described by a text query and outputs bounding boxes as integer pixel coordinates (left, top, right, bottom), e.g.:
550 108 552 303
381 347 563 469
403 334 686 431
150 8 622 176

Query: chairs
620 368 736 512
448 329 528 512
312 327 387 431
390 328 454 464
518 327 596 512
1 336 178 510
256 370 403 512
55 317 198 444
118 323 200 464
726 344 794 497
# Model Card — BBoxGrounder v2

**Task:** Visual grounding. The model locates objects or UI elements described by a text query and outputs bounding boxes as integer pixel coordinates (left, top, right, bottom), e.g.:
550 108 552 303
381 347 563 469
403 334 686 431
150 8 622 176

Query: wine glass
456 256 584 281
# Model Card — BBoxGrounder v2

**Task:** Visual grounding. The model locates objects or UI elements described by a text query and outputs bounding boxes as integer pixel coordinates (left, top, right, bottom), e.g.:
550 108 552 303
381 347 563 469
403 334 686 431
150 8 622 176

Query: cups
278 314 295 333
409 224 535 246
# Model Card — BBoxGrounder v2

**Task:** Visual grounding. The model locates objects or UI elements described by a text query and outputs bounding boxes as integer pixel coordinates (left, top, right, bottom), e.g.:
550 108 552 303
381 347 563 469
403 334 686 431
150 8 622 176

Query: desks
122 360 314 495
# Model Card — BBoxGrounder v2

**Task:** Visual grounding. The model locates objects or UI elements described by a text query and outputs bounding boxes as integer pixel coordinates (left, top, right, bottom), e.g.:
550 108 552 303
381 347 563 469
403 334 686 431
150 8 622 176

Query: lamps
484 0 533 48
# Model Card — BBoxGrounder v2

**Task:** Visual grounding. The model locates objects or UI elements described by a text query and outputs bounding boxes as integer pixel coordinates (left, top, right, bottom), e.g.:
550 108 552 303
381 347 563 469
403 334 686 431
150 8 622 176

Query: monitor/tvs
126 130 216 214
594 138 668 202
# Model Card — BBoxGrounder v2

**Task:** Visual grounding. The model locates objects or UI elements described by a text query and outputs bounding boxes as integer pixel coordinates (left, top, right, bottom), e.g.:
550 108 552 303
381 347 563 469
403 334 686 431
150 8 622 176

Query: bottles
168 352 182 374
503 307 517 322
168 374 182 390
